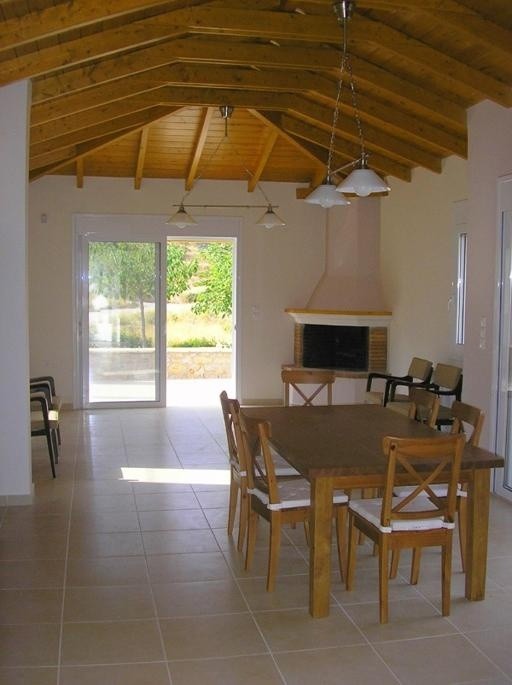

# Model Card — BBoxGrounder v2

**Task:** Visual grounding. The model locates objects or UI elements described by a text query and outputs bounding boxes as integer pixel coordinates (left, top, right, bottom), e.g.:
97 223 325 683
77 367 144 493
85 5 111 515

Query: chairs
356 392 436 559
388 403 486 585
221 393 312 551
30 376 63 479
366 357 435 406
241 413 347 592
344 433 467 624
389 368 466 433
281 366 351 505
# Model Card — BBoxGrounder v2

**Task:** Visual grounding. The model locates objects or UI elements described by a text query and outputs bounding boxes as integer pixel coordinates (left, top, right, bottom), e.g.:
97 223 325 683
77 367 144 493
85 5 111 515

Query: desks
241 403 505 621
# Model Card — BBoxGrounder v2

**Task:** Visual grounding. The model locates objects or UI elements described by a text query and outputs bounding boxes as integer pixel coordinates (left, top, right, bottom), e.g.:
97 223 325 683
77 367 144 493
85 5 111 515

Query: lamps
304 0 394 209
165 111 287 230
